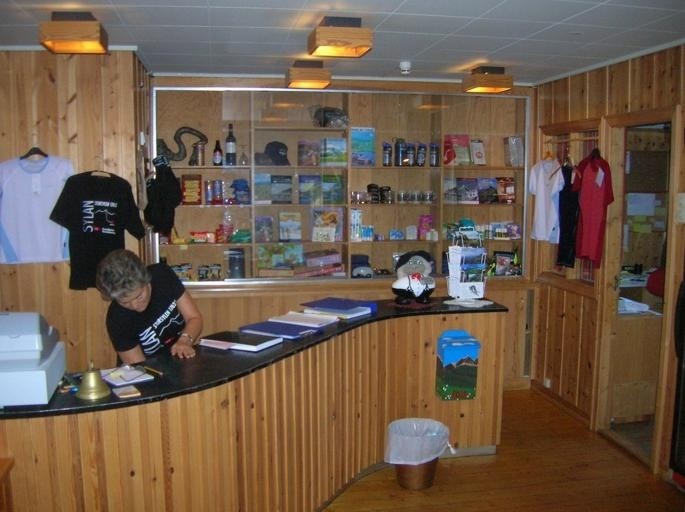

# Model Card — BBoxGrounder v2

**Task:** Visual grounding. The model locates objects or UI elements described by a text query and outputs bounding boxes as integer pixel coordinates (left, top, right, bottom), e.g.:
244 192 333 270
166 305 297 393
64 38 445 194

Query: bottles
191 141 206 166
351 182 434 205
225 123 237 166
212 138 223 166
350 208 374 242
382 136 439 166
477 224 509 239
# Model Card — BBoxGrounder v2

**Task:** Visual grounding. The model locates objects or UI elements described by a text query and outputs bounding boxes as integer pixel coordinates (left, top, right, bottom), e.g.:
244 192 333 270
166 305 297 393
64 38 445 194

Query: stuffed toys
391 250 436 305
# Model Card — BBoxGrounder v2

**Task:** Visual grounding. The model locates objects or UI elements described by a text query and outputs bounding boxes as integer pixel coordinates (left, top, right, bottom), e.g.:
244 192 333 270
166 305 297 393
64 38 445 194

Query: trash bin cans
386 418 449 490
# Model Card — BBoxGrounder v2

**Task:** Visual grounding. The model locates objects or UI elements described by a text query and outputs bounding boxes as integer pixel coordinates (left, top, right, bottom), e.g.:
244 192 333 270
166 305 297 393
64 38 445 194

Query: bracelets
181 333 193 344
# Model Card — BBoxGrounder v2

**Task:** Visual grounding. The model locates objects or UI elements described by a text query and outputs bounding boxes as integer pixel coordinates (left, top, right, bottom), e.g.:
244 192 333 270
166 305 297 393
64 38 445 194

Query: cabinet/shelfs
157 88 254 280
253 91 350 280
605 307 663 428
349 91 443 281
444 92 524 276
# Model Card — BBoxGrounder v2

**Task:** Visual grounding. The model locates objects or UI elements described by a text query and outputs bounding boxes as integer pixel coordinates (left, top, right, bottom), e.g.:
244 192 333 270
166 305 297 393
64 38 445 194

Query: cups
425 227 438 240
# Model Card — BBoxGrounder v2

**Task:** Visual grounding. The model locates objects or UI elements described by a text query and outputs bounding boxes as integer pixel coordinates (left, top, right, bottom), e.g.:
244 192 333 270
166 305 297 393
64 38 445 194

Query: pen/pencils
142 364 163 376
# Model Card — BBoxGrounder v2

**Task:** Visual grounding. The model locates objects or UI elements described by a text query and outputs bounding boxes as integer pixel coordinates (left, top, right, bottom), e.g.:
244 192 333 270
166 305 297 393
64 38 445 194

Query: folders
299 297 377 321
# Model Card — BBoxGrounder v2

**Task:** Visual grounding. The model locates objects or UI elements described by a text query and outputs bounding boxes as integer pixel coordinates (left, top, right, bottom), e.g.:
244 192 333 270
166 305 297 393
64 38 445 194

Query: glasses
111 363 147 381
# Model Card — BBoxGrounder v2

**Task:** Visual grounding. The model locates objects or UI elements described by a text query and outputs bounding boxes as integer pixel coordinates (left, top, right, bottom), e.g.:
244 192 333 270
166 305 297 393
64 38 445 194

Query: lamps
36 8 111 55
457 62 511 95
302 13 376 58
283 58 335 92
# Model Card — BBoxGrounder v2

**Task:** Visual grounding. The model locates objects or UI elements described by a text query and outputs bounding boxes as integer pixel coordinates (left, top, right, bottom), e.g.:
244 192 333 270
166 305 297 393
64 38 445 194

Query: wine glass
238 144 249 165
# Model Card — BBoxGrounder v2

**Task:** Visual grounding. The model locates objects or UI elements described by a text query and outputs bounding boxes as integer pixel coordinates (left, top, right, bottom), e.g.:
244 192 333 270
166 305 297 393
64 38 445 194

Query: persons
94 248 204 365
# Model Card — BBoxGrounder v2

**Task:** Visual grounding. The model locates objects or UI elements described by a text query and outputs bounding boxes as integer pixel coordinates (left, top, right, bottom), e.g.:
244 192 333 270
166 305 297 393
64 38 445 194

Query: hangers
542 140 603 180
89 154 117 178
18 133 48 161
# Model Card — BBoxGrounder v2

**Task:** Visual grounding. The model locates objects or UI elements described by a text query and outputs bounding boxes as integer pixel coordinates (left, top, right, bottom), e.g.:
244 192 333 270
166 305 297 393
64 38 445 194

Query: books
239 320 323 340
101 367 156 387
267 310 340 327
255 248 342 277
299 296 377 319
200 331 284 352
